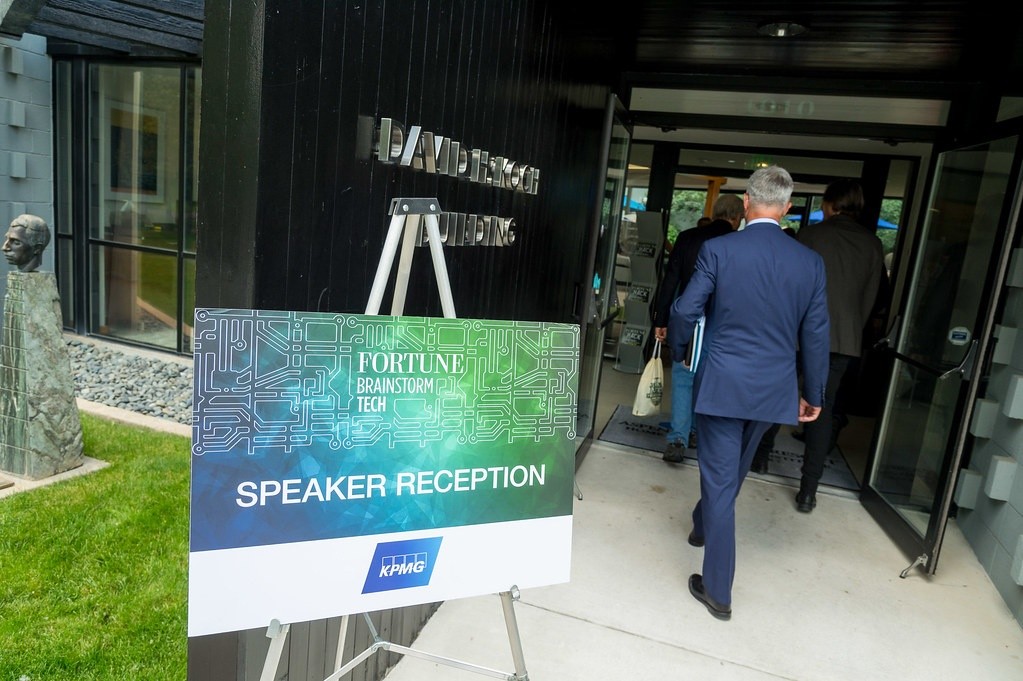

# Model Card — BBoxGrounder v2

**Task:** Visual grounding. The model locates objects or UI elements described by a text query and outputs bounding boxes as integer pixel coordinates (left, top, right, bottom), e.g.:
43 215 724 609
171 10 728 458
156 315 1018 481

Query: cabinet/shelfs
612 210 670 374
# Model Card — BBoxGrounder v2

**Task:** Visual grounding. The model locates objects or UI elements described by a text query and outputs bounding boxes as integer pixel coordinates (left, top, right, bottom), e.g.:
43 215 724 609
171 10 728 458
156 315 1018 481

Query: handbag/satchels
632 338 664 416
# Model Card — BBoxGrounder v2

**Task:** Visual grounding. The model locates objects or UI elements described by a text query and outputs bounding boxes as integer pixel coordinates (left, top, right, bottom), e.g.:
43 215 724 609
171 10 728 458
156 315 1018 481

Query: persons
0 213 51 272
664 164 830 621
654 177 891 514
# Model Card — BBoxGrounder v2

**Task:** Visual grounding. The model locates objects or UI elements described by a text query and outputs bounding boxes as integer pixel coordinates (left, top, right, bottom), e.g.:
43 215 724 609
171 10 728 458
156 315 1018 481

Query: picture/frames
103 98 167 206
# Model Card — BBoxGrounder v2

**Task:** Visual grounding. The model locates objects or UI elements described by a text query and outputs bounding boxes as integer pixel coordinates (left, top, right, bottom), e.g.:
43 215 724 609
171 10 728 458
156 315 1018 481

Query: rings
655 334 657 339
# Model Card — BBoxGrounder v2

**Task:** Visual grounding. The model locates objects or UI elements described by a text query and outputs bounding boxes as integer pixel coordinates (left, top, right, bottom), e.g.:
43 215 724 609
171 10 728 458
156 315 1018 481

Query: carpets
597 403 864 492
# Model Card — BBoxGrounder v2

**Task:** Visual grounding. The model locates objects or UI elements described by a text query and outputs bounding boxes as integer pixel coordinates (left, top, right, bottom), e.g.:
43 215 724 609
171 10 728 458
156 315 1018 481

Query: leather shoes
752 460 768 474
795 492 817 511
689 574 731 620
688 529 702 547
793 432 832 457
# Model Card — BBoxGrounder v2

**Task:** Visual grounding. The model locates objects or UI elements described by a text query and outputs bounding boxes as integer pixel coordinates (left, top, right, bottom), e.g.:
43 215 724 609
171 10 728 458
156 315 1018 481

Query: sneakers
663 443 684 463
